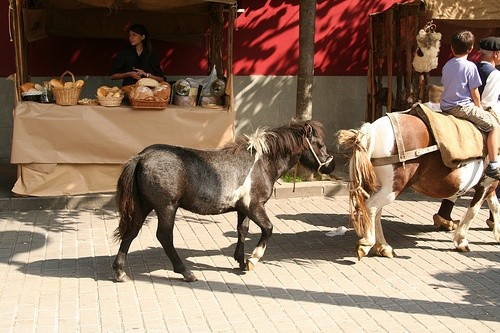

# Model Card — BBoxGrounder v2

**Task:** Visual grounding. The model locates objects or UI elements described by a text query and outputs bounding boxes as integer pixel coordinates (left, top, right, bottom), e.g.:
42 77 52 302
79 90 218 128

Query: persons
111 23 166 86
433 36 500 231
442 30 500 180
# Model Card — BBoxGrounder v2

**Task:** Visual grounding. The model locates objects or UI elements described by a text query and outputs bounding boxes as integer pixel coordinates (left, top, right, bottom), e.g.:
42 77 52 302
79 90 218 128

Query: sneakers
484 165 500 181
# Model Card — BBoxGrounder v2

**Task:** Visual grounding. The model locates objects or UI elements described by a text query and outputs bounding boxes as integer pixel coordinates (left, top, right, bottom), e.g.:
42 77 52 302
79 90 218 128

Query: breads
20 83 43 93
122 77 170 101
50 78 85 88
97 85 122 98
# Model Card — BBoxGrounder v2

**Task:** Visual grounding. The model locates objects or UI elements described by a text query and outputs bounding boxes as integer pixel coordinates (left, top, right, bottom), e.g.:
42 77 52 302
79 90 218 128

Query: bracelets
146 73 151 78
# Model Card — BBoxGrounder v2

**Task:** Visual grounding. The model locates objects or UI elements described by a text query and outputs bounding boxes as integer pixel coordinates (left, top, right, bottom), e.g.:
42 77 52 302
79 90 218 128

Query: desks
10 101 236 196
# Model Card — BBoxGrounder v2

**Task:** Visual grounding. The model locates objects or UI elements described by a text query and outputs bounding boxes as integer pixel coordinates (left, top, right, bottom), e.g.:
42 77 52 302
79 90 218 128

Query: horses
333 101 500 260
112 117 336 283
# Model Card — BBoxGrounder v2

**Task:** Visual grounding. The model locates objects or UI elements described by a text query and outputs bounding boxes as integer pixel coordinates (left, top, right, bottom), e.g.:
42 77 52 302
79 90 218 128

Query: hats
480 36 500 51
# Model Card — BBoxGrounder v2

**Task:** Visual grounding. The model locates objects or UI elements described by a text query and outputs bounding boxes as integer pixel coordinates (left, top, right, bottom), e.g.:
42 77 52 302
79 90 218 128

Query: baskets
96 95 124 107
52 71 82 106
131 99 169 109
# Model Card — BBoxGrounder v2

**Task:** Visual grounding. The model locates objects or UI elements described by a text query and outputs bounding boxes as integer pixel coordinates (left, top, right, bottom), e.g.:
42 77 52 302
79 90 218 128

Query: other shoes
486 220 494 229
433 213 461 231
